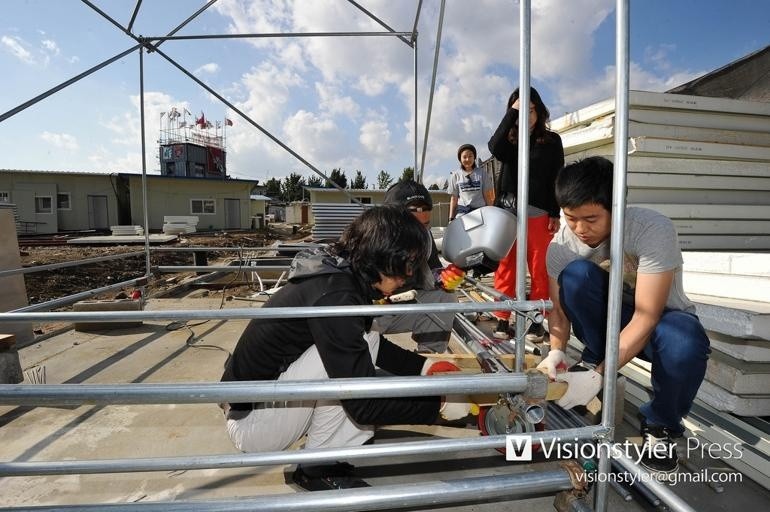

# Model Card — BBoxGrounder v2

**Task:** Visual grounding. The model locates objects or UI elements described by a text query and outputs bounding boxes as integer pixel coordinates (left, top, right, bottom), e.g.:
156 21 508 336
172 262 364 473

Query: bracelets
448 217 455 221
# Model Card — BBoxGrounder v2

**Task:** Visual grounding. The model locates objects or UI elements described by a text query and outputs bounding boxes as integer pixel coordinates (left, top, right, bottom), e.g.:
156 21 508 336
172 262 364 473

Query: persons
488 86 566 340
448 143 495 223
218 202 483 498
535 156 712 483
353 181 464 355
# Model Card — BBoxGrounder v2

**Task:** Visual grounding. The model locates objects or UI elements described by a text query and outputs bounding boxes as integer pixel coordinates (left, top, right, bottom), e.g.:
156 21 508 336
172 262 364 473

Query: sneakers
640 420 679 481
493 319 510 340
526 322 545 343
568 360 597 372
292 462 372 492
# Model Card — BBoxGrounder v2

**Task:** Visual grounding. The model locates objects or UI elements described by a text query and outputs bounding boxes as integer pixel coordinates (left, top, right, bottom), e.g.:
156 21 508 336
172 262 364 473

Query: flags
159 107 235 133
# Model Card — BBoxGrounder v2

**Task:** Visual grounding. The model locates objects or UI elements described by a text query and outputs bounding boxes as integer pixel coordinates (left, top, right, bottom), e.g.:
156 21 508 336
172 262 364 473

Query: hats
384 181 431 213
458 144 477 161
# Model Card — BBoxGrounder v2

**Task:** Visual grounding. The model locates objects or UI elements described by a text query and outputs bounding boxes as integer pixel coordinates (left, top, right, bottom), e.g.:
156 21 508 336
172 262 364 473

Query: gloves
440 264 465 291
440 394 480 421
555 370 603 411
536 350 568 381
420 359 462 376
